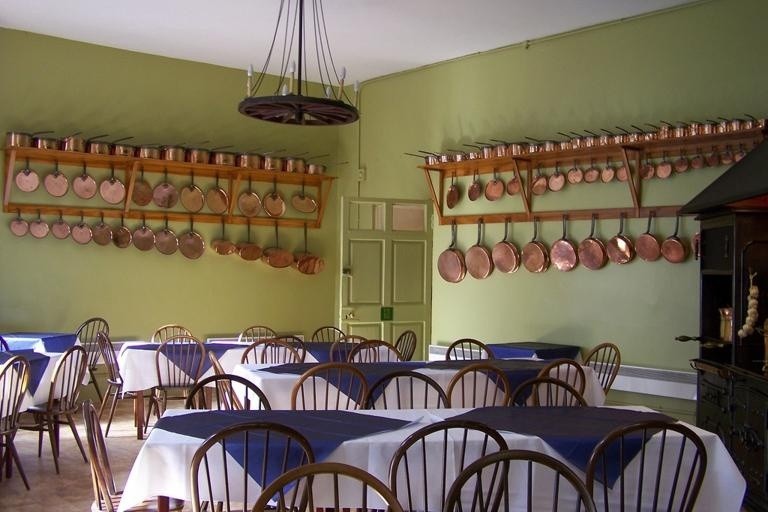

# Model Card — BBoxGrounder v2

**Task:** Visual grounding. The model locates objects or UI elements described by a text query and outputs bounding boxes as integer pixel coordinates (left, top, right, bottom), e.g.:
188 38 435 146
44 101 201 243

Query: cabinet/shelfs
674 136 767 512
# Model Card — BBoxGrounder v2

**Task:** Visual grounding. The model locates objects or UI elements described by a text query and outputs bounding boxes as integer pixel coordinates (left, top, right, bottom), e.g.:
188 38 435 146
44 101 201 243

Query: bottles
718 308 732 346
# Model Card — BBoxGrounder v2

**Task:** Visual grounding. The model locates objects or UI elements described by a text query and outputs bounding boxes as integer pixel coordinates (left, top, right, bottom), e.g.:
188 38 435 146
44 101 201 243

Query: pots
5 129 329 276
403 113 765 284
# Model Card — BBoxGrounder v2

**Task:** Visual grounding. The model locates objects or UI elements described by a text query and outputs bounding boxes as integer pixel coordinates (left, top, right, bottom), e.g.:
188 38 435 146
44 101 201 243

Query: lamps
236 0 362 127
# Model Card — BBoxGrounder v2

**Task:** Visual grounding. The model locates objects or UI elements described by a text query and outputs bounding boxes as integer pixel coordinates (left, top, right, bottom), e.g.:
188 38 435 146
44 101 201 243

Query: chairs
0 318 746 511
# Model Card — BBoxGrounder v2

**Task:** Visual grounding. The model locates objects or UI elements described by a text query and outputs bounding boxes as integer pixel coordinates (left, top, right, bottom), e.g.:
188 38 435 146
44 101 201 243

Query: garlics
737 284 760 340
